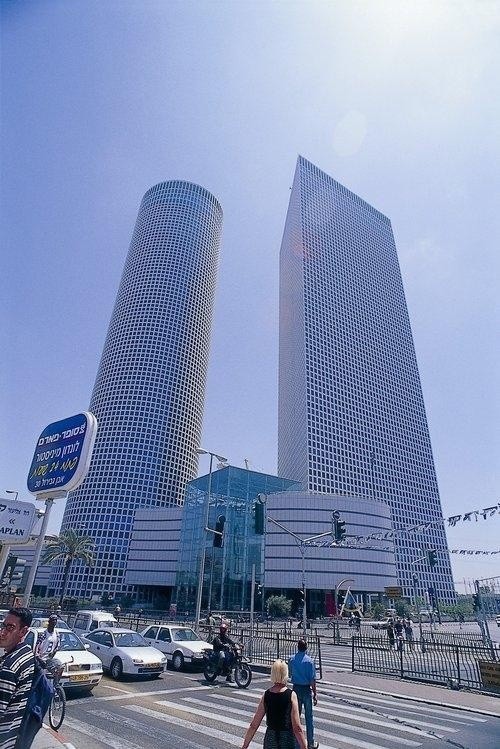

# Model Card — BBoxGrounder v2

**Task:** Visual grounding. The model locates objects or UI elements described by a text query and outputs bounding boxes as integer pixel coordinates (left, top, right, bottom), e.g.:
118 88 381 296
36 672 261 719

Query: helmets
219 623 228 630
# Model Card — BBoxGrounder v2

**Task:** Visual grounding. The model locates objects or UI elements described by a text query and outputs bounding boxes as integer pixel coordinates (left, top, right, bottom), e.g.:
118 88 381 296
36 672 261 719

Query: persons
114 604 121 615
206 613 216 638
211 622 244 684
0 606 36 749
288 638 318 749
241 658 308 749
33 613 65 688
386 611 415 652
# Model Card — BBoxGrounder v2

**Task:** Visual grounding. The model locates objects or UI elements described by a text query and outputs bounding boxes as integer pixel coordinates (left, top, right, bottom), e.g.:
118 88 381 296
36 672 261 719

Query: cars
195 613 233 627
370 617 412 629
0 603 213 692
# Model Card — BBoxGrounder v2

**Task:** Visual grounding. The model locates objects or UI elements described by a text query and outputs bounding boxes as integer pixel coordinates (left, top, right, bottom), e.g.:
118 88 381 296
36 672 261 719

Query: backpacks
16 658 56 749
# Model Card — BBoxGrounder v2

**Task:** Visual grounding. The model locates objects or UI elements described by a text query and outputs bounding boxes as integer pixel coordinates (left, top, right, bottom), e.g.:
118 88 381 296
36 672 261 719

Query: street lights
191 446 226 633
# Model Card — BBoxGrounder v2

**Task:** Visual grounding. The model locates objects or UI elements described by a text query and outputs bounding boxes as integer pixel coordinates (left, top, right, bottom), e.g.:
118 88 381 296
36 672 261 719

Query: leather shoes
307 741 319 749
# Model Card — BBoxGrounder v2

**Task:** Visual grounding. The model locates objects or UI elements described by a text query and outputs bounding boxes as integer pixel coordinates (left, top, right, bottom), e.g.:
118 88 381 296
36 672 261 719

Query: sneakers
226 677 235 683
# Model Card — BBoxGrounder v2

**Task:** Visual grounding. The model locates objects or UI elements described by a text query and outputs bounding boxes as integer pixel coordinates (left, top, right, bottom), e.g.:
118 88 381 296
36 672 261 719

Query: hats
48 614 57 622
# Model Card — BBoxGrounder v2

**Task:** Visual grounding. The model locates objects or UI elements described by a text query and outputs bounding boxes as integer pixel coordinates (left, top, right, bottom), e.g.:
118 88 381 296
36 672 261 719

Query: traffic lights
251 500 264 535
332 520 347 544
428 549 439 566
297 590 305 610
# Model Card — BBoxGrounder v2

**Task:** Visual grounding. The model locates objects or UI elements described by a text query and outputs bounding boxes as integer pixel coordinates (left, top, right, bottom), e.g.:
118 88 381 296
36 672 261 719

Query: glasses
0 621 16 632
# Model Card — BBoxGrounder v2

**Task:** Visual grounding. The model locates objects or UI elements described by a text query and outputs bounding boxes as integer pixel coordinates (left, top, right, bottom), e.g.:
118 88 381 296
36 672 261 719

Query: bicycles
41 657 74 730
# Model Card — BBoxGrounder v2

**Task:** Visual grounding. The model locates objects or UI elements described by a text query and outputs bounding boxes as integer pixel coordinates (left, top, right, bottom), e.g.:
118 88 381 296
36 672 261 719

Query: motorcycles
202 644 251 688
